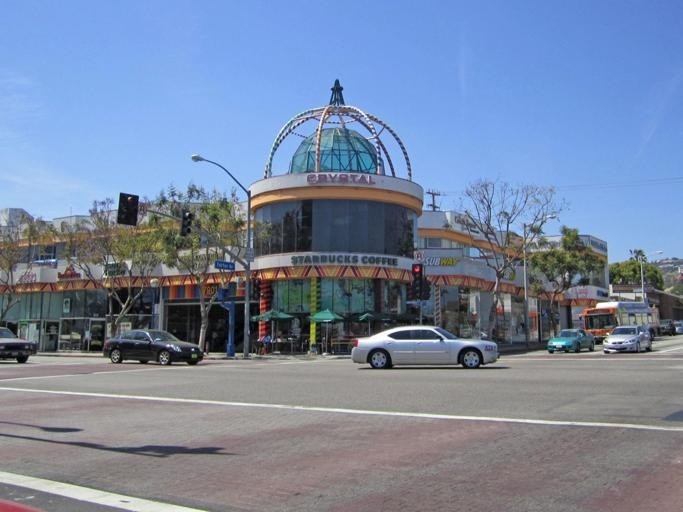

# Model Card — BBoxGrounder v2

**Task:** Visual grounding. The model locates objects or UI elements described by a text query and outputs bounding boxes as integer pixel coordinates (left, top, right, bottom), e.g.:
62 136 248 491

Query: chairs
248 335 354 357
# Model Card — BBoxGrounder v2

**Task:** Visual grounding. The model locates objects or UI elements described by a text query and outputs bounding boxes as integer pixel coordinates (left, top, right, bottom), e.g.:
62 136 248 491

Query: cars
102 328 203 366
380 311 559 343
0 327 37 363
546 328 595 354
350 325 499 369
60 337 102 350
658 318 682 336
602 325 652 354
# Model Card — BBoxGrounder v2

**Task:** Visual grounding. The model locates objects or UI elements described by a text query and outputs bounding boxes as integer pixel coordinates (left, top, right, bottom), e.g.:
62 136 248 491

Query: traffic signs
214 260 235 270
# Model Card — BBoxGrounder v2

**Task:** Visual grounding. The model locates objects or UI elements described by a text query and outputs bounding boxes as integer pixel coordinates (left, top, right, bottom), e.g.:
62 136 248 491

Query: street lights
640 250 663 302
523 214 556 350
190 154 251 357
149 277 158 327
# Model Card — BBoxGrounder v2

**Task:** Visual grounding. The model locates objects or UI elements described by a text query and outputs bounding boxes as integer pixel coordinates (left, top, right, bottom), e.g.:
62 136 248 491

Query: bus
578 302 660 345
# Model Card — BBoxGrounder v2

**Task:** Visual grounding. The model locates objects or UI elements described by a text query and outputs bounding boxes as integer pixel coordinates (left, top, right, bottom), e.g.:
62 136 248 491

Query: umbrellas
349 309 393 338
396 311 428 324
250 308 294 353
304 306 345 355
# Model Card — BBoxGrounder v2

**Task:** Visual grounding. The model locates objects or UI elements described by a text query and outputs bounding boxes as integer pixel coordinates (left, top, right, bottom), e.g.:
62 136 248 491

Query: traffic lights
179 210 192 237
411 264 422 299
117 193 138 226
